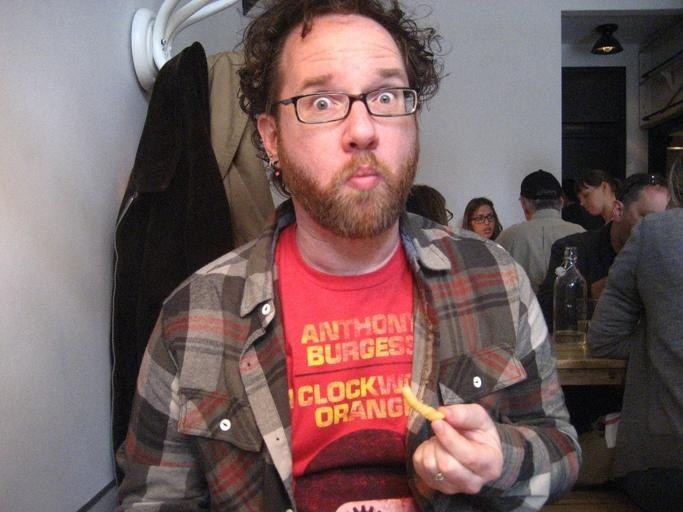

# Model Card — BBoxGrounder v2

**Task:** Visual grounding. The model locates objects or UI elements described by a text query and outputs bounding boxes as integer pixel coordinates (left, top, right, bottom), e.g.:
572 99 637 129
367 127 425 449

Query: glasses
469 213 497 224
272 86 418 124
444 206 453 222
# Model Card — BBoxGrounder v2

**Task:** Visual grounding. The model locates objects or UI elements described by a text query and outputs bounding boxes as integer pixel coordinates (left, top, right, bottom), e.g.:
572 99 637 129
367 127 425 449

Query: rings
428 473 444 480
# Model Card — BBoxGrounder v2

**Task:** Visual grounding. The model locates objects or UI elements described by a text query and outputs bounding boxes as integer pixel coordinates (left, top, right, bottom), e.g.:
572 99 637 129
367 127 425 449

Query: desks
528 343 627 388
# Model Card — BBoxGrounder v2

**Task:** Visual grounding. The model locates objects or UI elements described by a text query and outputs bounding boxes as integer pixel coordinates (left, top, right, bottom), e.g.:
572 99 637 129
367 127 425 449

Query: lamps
589 22 625 55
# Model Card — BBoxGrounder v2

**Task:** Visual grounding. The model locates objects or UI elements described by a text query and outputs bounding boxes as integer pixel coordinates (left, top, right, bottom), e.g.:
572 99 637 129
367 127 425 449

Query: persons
116 0 583 510
404 163 672 336
579 147 683 510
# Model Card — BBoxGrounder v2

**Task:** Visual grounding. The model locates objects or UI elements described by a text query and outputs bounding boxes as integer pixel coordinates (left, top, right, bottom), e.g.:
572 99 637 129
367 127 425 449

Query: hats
520 170 565 202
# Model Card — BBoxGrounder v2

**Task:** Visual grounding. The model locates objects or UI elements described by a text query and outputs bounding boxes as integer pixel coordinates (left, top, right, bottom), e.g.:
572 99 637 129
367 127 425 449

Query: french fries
402 387 445 421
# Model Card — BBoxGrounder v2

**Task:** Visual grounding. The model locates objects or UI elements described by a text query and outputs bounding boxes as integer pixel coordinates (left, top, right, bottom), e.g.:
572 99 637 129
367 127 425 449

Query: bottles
550 243 588 346
